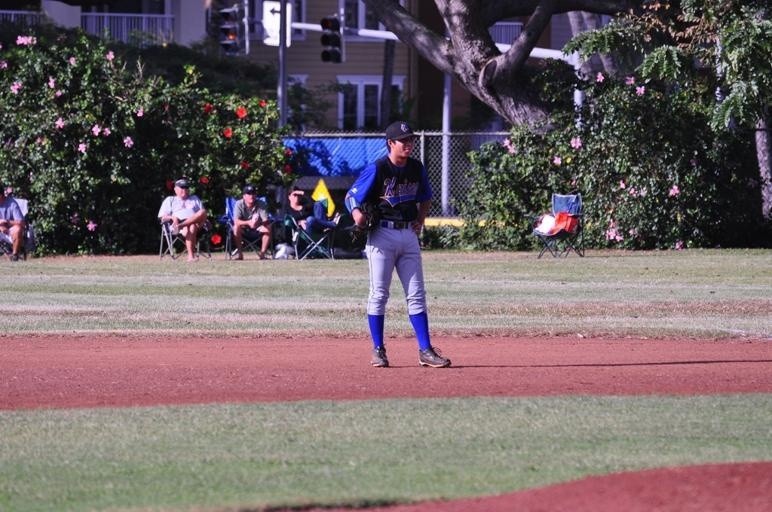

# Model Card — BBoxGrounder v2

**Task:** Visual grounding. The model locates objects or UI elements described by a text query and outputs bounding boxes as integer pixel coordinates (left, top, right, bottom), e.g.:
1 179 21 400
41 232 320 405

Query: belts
381 221 409 229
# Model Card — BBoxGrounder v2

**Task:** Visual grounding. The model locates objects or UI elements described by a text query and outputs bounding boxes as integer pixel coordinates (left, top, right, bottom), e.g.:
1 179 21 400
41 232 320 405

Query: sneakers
419 348 450 368
372 347 389 366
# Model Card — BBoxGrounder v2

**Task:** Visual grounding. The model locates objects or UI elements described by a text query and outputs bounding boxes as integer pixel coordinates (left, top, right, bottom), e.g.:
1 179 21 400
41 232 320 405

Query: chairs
530 192 592 260
282 196 338 265
220 195 280 263
150 193 218 261
0 196 37 260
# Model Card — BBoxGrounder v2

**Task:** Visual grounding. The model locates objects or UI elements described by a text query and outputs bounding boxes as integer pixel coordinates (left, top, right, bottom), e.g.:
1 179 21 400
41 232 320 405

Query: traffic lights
321 15 343 65
220 4 242 57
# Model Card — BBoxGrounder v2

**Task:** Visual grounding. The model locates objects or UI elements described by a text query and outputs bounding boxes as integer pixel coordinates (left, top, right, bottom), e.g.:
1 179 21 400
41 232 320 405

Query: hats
386 122 417 140
175 179 191 188
243 186 256 194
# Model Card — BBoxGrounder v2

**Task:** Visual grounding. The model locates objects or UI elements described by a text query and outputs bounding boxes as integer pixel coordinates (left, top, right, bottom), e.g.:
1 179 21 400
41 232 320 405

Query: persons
0 184 27 262
231 184 272 259
345 119 453 367
283 186 344 233
158 179 207 261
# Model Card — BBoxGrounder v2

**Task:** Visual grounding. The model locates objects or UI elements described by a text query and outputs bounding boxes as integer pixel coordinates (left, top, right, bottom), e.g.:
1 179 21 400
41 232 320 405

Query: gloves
351 225 367 251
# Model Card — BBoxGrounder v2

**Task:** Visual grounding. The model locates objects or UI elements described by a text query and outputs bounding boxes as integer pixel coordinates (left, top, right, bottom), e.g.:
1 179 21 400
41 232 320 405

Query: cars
469 116 555 160
